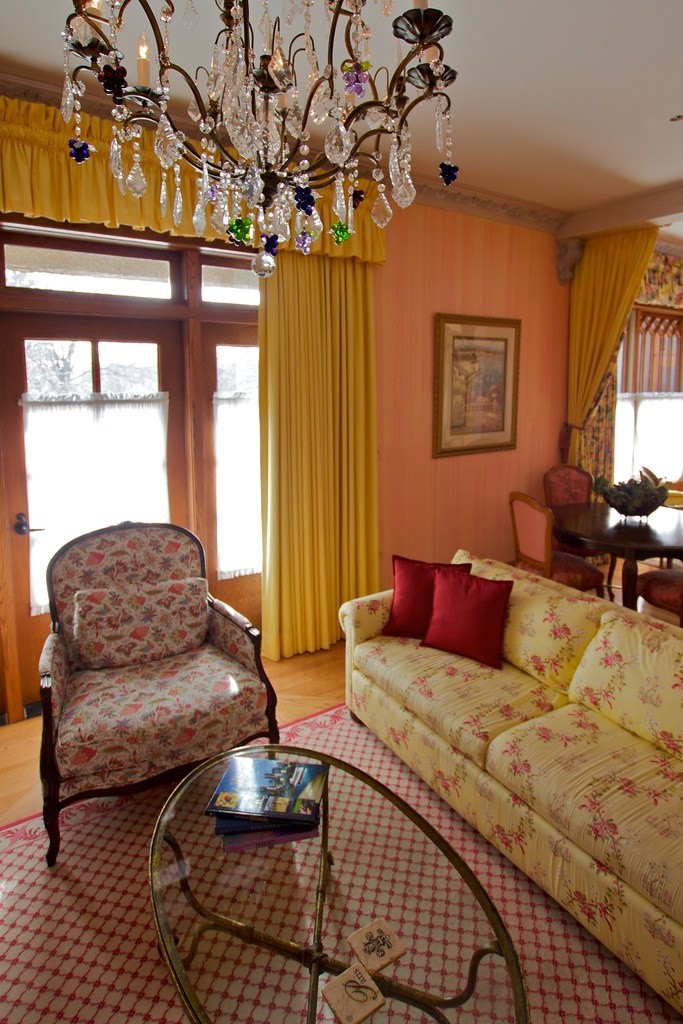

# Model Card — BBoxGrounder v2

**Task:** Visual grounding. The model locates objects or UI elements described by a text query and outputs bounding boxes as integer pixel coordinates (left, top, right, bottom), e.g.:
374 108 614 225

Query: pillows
70 577 211 671
419 566 514 671
383 555 473 640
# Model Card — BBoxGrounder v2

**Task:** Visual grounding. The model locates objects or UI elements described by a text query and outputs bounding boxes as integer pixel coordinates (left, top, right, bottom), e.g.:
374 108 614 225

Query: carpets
0 702 683 1024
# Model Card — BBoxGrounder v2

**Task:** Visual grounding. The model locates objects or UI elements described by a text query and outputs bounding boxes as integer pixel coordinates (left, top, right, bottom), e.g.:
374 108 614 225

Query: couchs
339 550 683 1017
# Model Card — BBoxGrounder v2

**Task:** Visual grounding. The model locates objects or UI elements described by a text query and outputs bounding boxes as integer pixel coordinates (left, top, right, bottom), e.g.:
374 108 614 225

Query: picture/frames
432 312 522 458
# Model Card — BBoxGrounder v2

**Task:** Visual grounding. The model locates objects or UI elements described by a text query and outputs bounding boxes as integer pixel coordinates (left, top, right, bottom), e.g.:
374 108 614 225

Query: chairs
543 464 617 602
637 568 683 628
38 520 281 867
508 491 604 598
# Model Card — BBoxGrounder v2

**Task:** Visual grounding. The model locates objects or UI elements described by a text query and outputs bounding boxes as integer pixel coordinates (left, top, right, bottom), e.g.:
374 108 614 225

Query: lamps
60 0 458 278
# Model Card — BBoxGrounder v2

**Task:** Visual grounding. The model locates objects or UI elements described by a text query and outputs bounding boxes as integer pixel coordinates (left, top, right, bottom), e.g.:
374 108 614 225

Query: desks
552 501 683 611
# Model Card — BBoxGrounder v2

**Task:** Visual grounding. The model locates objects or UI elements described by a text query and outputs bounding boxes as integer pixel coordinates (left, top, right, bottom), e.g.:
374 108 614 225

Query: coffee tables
149 744 529 1024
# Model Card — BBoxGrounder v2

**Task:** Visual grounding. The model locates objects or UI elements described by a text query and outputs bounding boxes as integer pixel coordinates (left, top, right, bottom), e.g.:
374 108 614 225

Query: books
214 816 320 833
205 756 330 824
222 830 321 850
